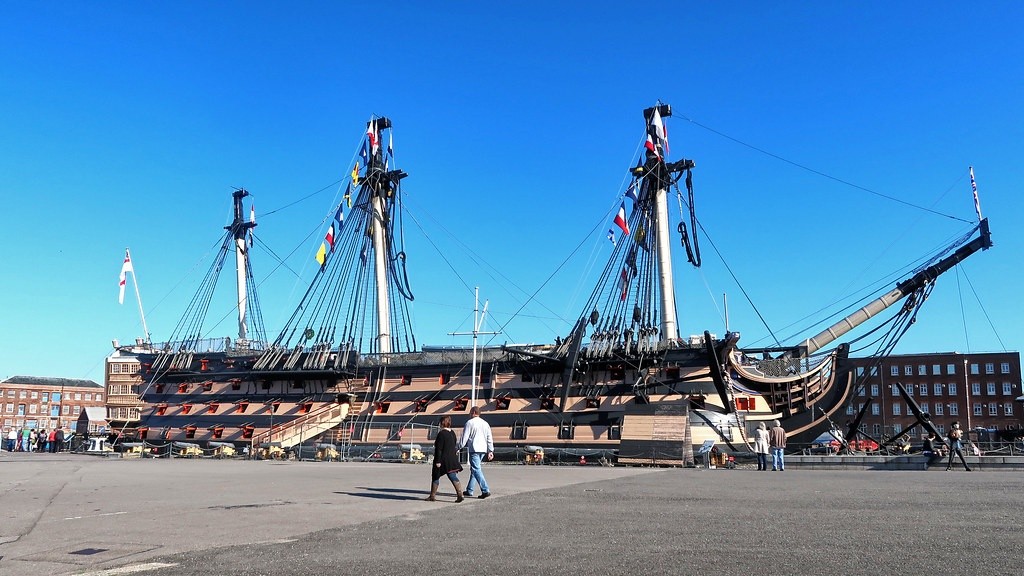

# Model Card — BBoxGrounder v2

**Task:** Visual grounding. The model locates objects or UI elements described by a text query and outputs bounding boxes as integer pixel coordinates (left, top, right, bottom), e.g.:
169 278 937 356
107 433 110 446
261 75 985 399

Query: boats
68 98 1003 469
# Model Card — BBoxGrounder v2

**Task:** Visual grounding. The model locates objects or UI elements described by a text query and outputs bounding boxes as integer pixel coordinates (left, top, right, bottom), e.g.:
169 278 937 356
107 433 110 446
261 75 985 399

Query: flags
359 138 368 166
334 203 344 230
384 154 389 172
343 181 351 208
365 120 374 148
351 162 359 186
605 107 670 301
324 220 335 253
613 200 630 235
118 252 132 305
315 243 327 275
372 123 379 156
387 133 393 158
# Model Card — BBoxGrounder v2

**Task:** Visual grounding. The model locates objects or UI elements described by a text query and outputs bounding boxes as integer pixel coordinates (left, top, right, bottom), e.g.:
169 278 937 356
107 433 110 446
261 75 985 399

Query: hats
759 422 766 430
773 420 780 427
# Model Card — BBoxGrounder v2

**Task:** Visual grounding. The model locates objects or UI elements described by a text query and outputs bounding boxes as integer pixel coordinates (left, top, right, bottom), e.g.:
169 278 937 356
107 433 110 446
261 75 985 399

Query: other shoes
780 469 784 471
923 463 929 470
772 468 777 471
946 467 951 471
757 468 766 471
965 467 971 471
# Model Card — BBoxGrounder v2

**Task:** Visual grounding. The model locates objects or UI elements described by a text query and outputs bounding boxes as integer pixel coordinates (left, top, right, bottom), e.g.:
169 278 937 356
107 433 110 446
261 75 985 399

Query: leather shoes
462 490 472 496
477 491 490 499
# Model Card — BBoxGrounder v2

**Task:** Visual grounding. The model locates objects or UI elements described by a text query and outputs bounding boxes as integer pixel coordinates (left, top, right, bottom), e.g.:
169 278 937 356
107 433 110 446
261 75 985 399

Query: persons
6 426 64 454
424 415 465 503
770 419 787 471
753 422 770 471
945 421 972 471
455 406 495 500
922 432 940 471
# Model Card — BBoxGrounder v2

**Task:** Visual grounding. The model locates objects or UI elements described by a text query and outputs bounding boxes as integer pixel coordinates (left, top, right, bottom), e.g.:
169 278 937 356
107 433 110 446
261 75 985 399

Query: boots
451 480 464 503
424 481 438 501
